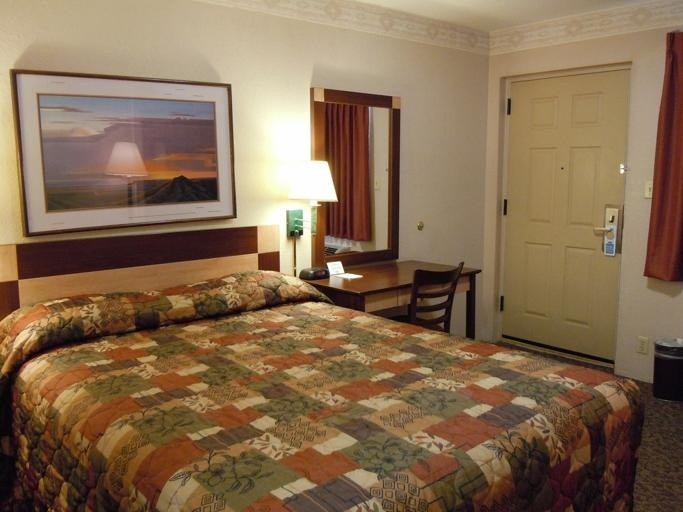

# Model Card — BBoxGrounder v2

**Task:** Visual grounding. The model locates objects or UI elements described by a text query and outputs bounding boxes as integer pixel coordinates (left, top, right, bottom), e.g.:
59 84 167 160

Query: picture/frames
9 69 237 237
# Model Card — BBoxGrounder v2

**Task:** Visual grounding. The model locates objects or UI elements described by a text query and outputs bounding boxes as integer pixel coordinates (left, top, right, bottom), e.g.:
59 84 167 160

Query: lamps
288 160 339 280
104 142 147 206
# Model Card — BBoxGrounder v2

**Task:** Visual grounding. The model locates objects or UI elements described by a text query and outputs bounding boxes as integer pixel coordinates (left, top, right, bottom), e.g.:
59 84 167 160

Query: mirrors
310 87 401 269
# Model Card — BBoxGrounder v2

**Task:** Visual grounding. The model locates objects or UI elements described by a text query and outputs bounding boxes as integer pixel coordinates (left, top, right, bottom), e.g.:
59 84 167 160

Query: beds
1 225 644 512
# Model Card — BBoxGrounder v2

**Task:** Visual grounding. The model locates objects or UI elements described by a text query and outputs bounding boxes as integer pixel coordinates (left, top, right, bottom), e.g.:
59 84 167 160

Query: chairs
386 262 464 334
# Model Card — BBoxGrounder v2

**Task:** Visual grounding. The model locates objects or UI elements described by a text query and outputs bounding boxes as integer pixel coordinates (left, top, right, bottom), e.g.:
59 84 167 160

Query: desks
300 260 482 340
